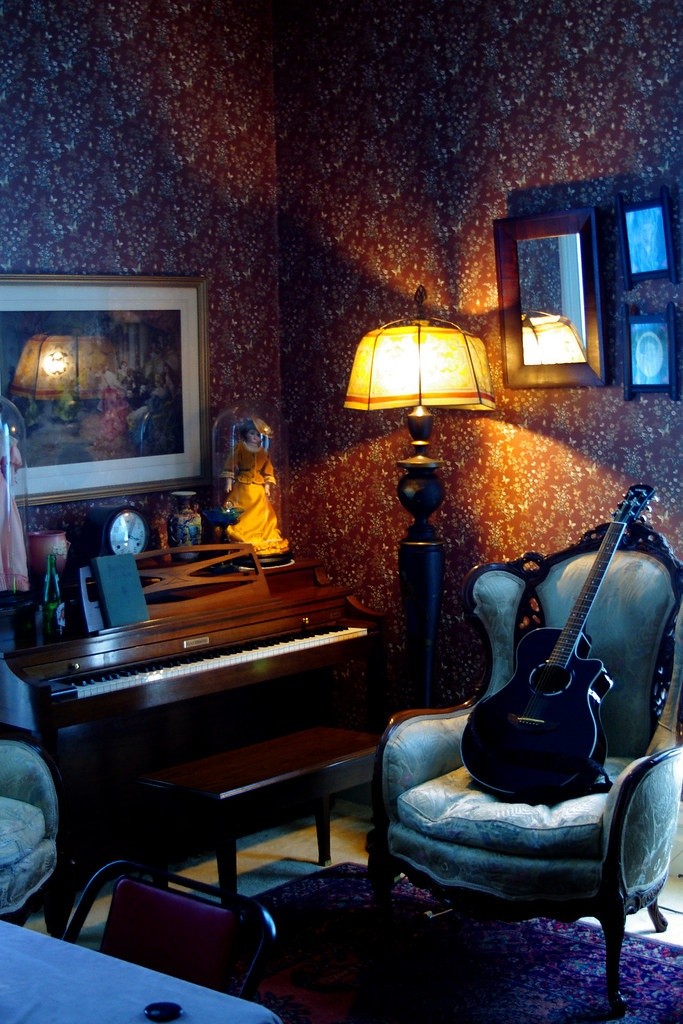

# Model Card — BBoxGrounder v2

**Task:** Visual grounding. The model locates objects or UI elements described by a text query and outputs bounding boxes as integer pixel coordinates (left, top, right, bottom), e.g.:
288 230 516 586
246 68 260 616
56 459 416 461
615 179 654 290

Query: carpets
69 857 683 1024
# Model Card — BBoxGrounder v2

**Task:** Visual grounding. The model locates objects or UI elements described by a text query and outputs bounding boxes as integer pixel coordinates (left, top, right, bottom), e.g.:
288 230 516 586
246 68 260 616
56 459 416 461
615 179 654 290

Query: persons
218 428 288 551
0 401 30 592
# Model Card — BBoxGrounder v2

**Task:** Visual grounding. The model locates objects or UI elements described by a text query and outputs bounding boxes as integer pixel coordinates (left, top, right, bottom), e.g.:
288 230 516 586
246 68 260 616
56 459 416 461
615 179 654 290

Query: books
93 554 151 627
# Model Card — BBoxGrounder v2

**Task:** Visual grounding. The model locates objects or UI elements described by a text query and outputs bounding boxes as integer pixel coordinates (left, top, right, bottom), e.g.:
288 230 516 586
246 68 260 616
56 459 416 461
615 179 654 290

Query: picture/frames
621 301 678 401
614 185 679 292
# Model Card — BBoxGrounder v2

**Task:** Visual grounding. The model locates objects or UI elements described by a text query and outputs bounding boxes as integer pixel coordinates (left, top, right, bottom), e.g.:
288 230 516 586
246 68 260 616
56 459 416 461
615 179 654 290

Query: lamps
9 319 129 464
521 309 587 365
342 284 499 735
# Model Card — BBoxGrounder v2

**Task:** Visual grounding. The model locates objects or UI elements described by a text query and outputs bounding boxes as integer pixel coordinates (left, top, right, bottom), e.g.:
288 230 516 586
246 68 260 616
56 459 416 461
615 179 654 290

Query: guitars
460 483 658 802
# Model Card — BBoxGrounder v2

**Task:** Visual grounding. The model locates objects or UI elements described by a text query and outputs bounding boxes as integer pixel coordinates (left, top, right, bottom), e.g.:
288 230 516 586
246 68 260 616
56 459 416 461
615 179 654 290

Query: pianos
1 554 401 939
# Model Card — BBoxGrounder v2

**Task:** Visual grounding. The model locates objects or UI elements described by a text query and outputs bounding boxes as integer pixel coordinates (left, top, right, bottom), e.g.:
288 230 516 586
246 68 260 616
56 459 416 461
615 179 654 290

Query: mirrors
0 273 212 508
492 206 605 389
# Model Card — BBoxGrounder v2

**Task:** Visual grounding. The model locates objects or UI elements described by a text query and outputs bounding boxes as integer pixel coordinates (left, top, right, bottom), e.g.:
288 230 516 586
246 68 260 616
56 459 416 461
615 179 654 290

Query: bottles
167 491 204 562
41 554 66 639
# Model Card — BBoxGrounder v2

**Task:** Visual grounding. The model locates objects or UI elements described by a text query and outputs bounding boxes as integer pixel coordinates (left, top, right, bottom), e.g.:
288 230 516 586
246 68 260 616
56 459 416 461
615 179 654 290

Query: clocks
101 506 150 556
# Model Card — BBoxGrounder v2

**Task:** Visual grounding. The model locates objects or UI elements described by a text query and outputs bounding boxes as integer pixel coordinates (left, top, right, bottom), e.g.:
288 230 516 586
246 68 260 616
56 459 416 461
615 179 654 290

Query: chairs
60 859 278 1000
0 731 70 939
365 512 683 1020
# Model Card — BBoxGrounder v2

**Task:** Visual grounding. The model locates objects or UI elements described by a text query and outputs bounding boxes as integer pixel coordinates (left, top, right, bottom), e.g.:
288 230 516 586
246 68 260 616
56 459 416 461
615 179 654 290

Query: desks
0 920 283 1024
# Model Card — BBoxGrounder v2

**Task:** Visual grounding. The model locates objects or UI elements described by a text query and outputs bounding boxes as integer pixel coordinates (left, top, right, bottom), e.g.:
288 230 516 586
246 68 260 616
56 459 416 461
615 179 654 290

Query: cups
28 529 70 576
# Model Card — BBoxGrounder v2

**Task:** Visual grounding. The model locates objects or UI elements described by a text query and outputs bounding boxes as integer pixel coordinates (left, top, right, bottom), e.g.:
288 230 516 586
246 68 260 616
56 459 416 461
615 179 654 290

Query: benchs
131 725 381 906
